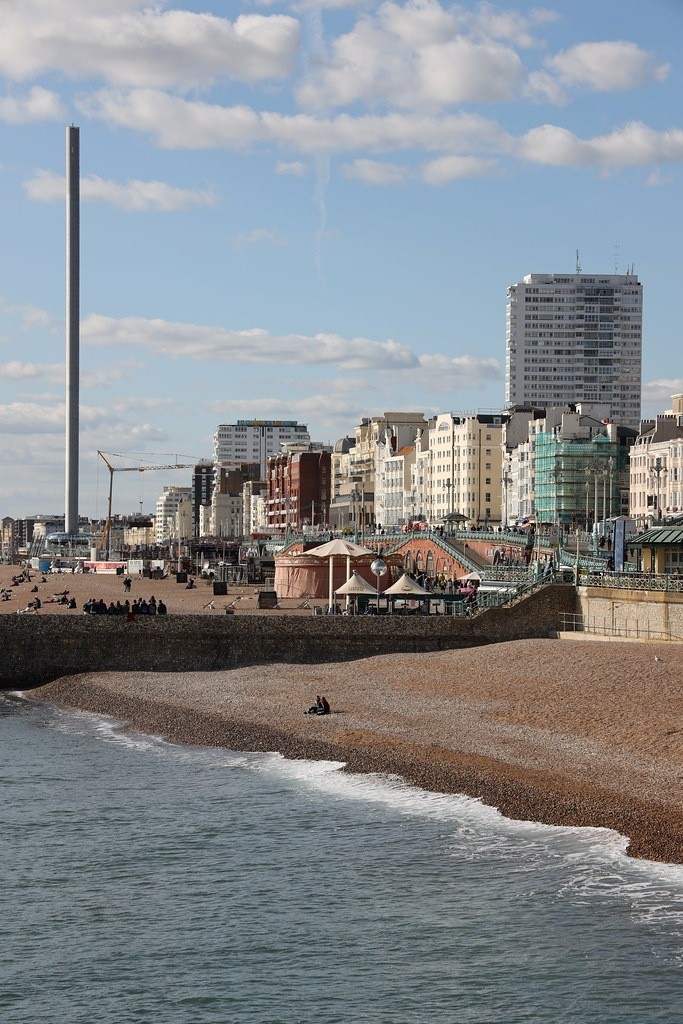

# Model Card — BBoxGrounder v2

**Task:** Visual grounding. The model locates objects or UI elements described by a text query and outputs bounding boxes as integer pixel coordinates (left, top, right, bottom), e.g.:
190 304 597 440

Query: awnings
625 530 683 548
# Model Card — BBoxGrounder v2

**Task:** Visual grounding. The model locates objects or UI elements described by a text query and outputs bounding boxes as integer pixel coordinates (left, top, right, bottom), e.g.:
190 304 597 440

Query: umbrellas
459 572 487 579
294 539 371 612
335 571 380 615
383 573 433 614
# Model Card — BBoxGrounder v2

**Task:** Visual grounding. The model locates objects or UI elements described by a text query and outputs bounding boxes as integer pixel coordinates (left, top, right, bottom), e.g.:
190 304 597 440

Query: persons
11 569 31 586
30 585 38 592
94 566 97 574
83 596 166 615
25 596 40 614
471 525 523 534
599 536 605 548
304 695 330 715
61 590 76 609
188 579 194 588
41 577 46 582
436 525 443 536
425 573 451 594
1 589 12 601
123 578 132 592
